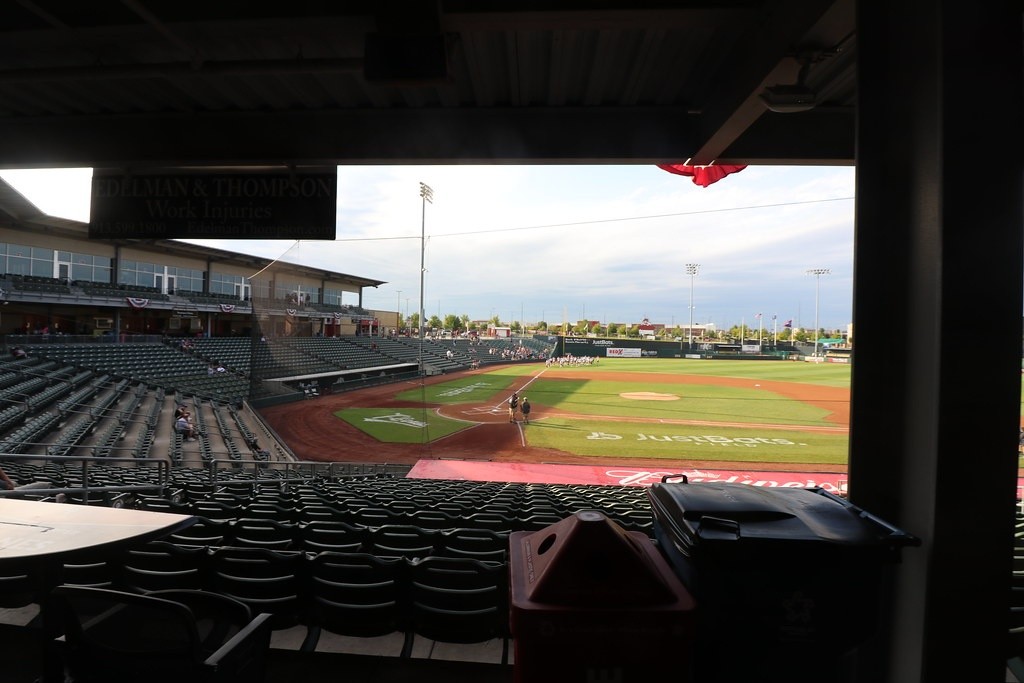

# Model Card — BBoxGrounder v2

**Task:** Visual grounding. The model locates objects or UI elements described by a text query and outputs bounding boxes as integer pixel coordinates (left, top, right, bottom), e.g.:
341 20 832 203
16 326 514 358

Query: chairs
0 273 1024 683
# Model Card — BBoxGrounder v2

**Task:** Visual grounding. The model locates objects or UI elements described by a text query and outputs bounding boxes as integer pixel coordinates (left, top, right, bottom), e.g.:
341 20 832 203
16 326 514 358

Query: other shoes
523 422 525 424
188 437 195 442
513 422 517 424
509 420 513 423
526 422 529 425
194 432 198 435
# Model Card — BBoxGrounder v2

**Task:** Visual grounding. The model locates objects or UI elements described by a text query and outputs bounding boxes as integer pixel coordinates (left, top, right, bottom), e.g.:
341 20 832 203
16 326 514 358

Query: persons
174 404 197 442
179 337 193 351
251 437 264 454
546 355 599 368
370 342 381 353
520 397 530 425
207 363 214 375
389 326 482 370
217 363 228 373
509 393 519 424
519 339 523 347
488 344 549 361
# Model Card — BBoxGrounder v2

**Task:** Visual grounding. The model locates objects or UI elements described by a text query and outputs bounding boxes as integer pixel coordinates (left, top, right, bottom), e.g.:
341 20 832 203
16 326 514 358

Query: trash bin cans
648 475 922 683
508 509 698 682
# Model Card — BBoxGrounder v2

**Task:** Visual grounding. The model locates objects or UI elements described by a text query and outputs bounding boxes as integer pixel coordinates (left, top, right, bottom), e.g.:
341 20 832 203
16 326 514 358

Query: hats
182 339 185 342
185 342 189 345
183 414 189 416
523 397 528 400
183 404 187 407
